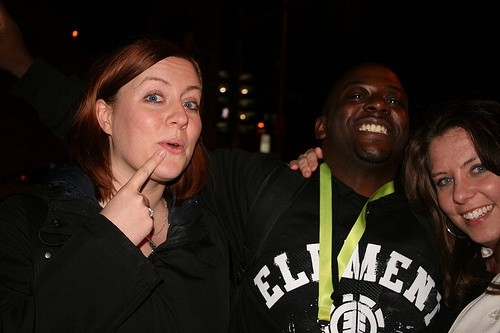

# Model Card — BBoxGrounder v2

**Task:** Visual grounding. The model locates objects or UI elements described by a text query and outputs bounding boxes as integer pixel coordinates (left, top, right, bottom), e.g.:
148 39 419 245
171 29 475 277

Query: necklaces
145 200 168 236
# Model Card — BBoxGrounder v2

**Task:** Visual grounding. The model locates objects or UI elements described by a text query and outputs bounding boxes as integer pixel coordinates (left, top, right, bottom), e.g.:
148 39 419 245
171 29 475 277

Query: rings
147 208 154 216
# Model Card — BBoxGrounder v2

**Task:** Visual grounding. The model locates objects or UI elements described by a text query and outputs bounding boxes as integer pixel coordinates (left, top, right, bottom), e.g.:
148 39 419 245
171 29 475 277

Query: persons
0 38 231 333
0 4 496 333
286 96 500 333
